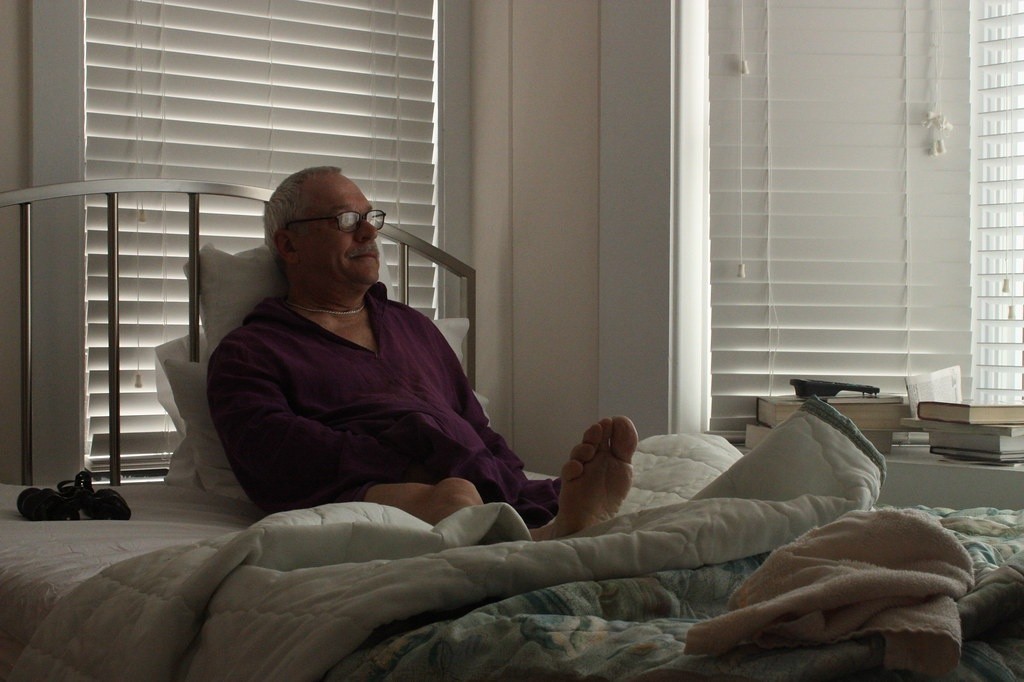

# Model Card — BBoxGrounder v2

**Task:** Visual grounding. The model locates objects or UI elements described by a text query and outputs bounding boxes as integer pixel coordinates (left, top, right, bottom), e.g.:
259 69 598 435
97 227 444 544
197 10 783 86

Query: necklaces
285 298 366 314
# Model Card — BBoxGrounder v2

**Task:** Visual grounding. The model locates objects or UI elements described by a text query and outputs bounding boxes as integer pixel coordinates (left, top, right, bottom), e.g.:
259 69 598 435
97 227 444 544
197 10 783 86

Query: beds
0 179 1024 682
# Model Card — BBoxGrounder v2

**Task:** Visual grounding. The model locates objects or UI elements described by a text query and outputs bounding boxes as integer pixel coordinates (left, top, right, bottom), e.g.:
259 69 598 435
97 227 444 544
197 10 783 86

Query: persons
207 166 635 551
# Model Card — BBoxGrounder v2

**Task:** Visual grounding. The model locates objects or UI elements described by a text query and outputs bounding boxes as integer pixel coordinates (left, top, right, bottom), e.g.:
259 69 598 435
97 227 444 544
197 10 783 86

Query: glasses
286 210 386 233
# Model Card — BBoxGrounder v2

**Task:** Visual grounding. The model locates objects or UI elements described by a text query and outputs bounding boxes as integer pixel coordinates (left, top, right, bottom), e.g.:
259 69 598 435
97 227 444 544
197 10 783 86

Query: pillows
155 316 469 496
182 244 287 355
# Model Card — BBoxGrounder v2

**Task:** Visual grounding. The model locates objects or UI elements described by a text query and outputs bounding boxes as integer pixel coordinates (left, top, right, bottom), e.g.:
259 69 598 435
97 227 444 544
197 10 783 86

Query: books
744 390 1024 467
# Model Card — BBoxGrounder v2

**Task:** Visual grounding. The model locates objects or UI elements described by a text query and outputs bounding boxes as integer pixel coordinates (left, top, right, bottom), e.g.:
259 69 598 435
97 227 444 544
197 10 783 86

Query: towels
682 510 973 673
